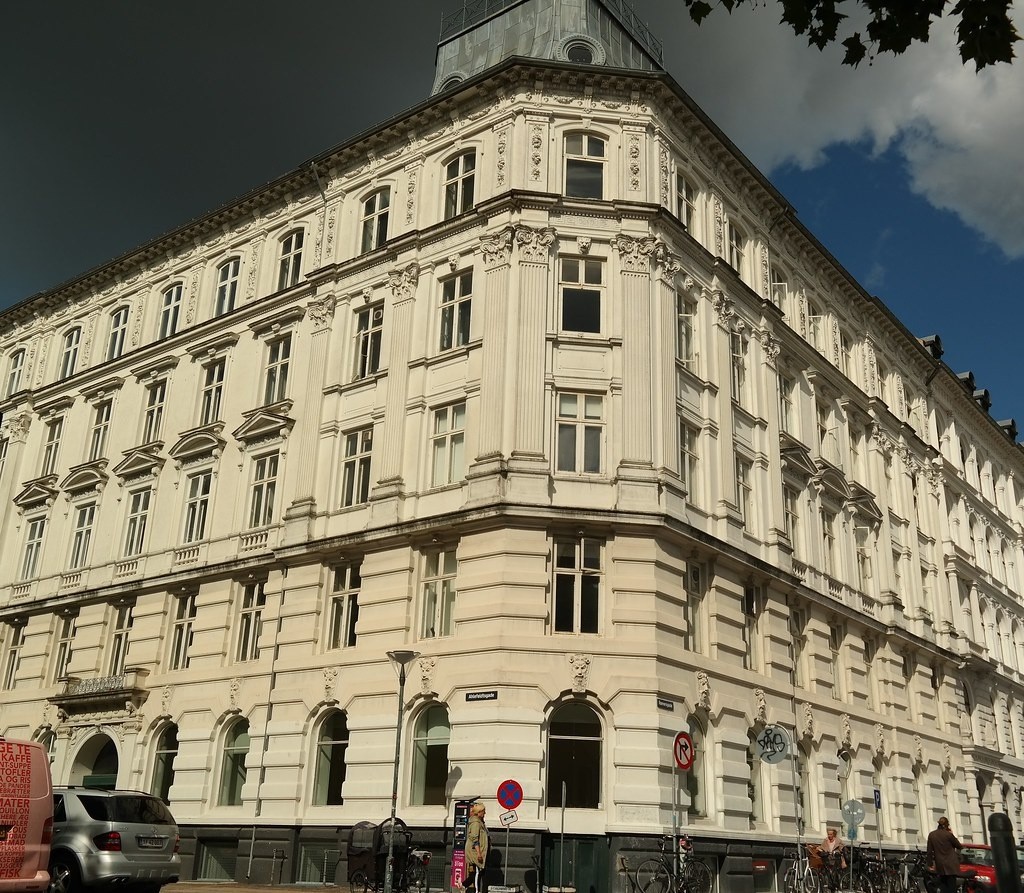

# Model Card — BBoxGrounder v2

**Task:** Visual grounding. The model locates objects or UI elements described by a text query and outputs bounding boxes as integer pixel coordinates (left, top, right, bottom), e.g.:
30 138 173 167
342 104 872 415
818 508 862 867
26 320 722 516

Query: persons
926 817 963 893
458 803 491 893
817 828 847 893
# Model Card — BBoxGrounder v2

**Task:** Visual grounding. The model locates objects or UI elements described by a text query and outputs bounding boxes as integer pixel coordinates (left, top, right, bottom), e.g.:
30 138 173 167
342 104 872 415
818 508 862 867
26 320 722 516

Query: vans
0 734 56 893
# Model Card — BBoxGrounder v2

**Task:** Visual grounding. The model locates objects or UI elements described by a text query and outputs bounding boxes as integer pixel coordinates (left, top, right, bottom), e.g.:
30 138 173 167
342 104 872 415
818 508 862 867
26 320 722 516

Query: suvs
50 784 182 893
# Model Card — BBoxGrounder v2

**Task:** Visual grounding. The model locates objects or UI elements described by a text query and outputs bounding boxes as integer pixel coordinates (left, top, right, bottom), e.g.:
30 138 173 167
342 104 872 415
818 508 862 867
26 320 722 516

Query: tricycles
346 816 430 893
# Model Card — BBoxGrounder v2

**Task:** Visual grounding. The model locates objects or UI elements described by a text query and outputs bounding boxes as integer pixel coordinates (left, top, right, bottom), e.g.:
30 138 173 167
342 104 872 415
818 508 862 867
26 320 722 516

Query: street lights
383 650 422 893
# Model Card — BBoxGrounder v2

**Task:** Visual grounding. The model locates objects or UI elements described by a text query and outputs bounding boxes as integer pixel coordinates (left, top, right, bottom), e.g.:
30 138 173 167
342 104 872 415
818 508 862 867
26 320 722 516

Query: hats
472 804 485 814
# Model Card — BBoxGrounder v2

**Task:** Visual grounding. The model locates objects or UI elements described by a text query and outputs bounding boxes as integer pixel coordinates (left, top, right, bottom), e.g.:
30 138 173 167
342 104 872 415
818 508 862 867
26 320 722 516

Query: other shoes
457 883 467 893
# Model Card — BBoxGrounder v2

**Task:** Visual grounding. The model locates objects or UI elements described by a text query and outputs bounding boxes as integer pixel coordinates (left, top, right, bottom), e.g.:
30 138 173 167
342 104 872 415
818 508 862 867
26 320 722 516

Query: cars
931 844 997 893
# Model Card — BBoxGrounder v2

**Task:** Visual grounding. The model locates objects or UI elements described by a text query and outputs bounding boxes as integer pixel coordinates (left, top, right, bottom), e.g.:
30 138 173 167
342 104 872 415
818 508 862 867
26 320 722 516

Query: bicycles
635 834 714 893
784 847 820 893
816 842 989 893
